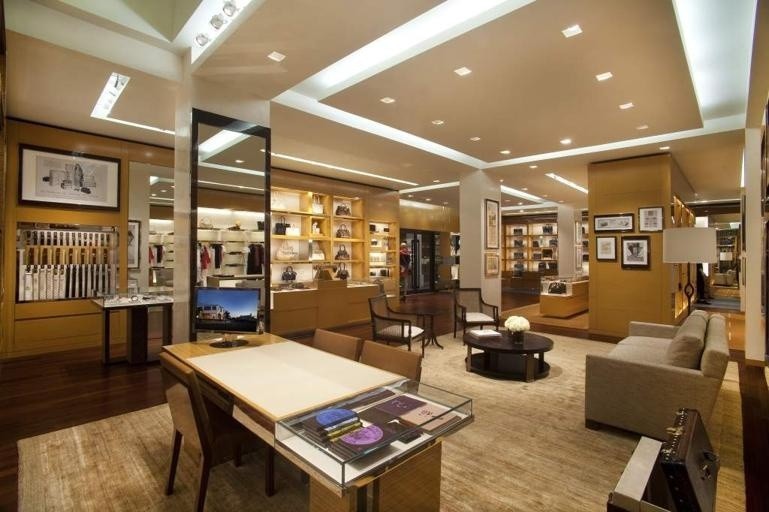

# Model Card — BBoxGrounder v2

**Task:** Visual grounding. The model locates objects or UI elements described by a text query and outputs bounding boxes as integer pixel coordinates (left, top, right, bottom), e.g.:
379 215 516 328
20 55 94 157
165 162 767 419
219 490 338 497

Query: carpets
17 321 641 511
499 302 588 329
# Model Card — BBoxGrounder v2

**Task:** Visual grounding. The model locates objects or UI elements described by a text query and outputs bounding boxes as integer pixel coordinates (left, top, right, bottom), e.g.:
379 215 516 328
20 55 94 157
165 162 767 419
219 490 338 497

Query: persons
127 230 134 265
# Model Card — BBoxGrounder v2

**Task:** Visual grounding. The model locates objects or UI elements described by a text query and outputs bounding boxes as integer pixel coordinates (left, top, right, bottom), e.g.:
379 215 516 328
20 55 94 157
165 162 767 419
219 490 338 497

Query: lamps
661 227 718 318
719 251 733 286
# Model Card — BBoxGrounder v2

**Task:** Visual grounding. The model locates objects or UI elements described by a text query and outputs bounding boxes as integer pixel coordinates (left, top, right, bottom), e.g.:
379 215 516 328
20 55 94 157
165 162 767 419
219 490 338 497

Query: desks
412 305 445 349
156 332 476 512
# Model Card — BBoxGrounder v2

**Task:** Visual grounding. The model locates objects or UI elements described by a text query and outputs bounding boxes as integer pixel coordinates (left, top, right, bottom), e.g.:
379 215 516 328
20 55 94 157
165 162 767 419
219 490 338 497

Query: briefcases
606 406 720 511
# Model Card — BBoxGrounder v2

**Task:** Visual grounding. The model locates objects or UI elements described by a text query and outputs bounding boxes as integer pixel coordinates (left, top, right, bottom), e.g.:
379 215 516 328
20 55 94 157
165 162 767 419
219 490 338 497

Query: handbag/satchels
548 282 566 295
312 204 324 214
336 250 350 261
282 266 297 280
275 223 290 235
336 206 352 216
337 229 351 238
311 249 325 261
336 269 349 279
276 247 293 261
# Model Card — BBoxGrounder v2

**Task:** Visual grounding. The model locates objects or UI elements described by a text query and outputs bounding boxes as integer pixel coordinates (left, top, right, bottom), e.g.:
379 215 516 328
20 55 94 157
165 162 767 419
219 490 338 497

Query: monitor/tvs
191 287 261 347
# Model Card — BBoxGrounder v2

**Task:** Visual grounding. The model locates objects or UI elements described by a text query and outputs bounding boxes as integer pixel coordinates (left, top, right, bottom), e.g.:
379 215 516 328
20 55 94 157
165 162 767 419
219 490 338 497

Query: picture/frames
593 213 634 233
115 219 141 269
621 235 651 268
486 198 500 250
18 141 121 212
638 206 664 232
596 236 617 261
484 253 499 277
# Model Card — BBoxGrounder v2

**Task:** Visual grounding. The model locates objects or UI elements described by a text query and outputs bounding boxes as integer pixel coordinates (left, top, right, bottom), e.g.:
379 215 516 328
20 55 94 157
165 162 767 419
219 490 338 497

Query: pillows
662 309 709 368
727 269 732 274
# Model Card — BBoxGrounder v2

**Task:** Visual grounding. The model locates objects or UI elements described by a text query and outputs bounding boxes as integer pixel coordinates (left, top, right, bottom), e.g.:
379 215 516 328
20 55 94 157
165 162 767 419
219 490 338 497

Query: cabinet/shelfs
149 178 398 296
501 218 589 276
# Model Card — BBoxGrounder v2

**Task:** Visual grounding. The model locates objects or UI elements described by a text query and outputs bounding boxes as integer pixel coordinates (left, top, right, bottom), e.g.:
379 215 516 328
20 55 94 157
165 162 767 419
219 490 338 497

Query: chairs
157 351 277 511
358 340 422 384
366 295 425 358
451 288 498 347
585 314 730 437
311 327 362 362
712 270 733 286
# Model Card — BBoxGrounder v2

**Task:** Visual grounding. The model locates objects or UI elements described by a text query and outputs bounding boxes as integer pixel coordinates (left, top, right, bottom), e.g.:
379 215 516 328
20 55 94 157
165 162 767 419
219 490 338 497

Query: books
301 408 397 464
